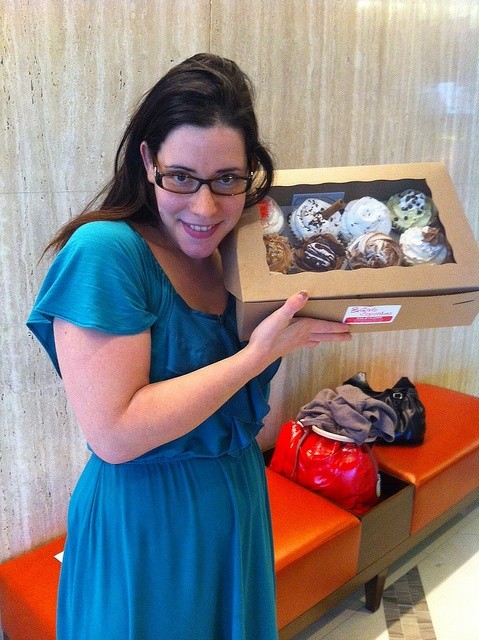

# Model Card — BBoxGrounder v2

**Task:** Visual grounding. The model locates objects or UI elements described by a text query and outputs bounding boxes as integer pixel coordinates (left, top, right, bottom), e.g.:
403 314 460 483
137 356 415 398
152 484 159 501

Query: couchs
1 383 479 640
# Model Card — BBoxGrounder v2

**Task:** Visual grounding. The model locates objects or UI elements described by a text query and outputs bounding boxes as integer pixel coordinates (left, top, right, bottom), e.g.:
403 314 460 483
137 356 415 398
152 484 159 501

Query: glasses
152 156 255 196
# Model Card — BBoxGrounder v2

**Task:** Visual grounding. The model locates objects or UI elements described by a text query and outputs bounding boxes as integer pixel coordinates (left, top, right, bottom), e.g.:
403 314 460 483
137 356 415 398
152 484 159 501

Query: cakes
258 192 286 234
287 193 346 241
345 229 402 267
397 223 448 265
342 191 392 242
262 236 292 275
386 188 438 228
294 232 347 271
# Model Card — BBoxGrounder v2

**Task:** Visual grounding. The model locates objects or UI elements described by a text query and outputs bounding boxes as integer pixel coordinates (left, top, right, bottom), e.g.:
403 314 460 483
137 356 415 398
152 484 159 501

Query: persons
26 52 352 640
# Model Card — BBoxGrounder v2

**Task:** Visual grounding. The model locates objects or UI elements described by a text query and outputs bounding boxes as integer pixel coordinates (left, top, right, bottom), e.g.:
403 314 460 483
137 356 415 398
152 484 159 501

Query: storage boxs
223 162 479 344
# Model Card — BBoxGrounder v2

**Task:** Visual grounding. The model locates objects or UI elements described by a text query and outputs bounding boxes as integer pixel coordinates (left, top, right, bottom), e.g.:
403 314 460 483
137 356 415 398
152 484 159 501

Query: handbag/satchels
343 372 425 446
270 420 382 515
